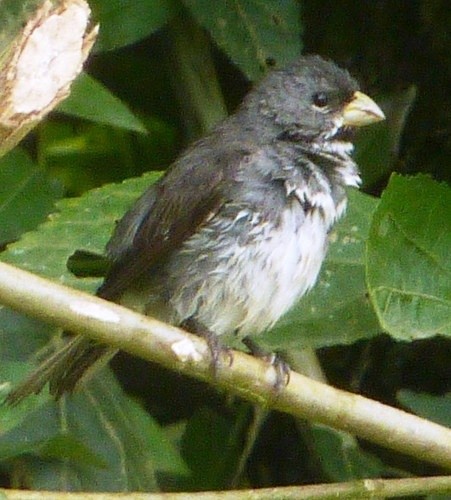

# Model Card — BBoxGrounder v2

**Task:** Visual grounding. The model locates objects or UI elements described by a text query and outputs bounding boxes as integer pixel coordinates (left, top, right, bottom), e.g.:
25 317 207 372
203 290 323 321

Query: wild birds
0 56 386 410
